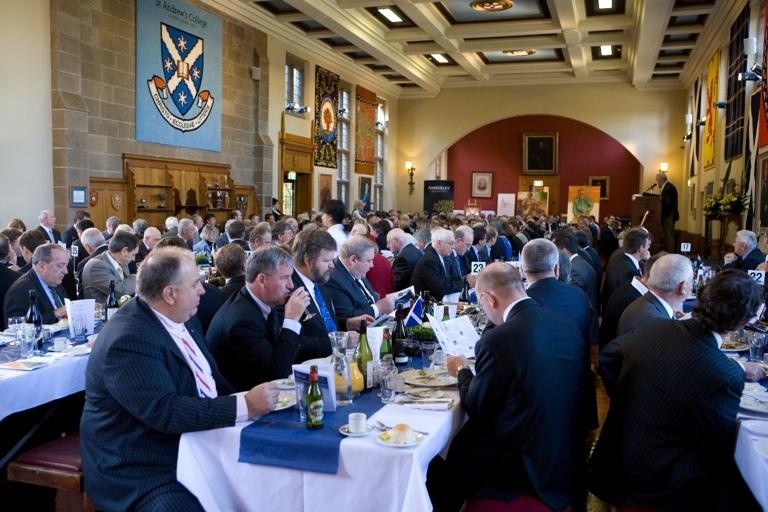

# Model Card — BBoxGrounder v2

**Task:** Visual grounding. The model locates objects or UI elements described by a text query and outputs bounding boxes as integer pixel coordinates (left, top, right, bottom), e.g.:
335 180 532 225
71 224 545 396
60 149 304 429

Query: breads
394 423 411 437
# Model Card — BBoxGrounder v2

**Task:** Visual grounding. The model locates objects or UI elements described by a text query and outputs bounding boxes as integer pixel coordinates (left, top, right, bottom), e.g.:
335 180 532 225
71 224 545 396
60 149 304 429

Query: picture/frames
523 131 559 174
589 176 611 202
471 171 494 199
752 151 768 235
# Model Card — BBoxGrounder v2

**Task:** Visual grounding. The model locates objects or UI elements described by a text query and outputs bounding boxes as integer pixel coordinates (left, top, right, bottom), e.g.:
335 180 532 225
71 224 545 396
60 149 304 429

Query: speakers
744 38 757 55
252 66 261 80
423 180 455 212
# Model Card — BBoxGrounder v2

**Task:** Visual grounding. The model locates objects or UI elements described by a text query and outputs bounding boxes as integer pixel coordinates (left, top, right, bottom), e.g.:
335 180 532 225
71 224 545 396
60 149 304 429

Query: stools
460 493 552 512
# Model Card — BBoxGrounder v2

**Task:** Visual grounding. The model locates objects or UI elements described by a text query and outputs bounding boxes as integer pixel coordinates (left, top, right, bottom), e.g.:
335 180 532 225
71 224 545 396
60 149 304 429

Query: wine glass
286 284 317 323
7 316 25 345
402 339 420 368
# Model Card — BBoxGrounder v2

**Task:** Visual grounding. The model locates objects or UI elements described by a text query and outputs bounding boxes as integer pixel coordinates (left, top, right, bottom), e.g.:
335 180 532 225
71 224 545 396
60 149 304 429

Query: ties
456 255 463 279
117 266 125 280
314 281 337 333
48 287 62 309
355 278 373 304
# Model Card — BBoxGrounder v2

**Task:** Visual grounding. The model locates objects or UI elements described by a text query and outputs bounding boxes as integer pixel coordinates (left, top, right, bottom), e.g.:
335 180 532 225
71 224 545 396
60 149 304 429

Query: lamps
406 160 415 193
740 38 762 79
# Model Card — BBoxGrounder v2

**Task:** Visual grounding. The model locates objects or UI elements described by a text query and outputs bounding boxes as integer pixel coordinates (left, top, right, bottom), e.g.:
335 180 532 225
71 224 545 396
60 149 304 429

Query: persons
656 172 679 253
0 197 767 512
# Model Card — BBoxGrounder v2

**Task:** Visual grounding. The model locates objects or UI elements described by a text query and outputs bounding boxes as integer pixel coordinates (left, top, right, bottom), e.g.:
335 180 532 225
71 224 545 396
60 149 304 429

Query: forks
377 421 427 436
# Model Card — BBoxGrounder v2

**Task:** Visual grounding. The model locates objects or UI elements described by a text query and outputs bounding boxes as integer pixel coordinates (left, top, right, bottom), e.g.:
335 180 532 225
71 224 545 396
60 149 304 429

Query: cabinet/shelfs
122 152 261 236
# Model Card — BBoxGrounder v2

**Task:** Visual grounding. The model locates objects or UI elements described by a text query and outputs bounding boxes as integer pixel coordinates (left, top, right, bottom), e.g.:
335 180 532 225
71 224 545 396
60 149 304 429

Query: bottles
743 320 767 334
379 327 392 360
421 290 432 322
441 306 450 322
224 191 230 209
352 319 374 392
693 258 705 293
105 279 117 321
327 330 351 402
23 289 42 346
213 182 224 210
392 303 409 367
302 364 325 430
458 277 470 305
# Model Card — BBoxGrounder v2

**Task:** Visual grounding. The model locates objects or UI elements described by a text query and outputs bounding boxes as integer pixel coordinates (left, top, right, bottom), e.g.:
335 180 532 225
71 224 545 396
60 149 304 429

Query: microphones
646 183 657 191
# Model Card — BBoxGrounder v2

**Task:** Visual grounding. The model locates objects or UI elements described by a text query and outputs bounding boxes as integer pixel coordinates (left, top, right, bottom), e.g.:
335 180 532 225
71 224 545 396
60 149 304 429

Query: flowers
703 159 754 214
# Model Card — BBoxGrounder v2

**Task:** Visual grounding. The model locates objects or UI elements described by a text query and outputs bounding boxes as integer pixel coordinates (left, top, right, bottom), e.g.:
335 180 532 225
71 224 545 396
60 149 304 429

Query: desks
464 205 481 218
704 211 743 264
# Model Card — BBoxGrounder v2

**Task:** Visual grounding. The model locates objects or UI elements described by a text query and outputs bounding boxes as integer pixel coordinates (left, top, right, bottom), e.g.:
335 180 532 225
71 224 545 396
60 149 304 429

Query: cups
421 341 435 368
297 385 308 423
53 337 67 349
19 325 36 357
374 361 405 404
68 313 87 341
347 413 366 433
748 331 768 362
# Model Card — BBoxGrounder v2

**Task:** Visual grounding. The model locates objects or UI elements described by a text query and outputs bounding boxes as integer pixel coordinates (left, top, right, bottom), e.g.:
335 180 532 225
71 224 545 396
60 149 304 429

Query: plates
752 439 767 461
46 346 73 352
741 420 767 436
398 370 458 388
745 359 768 378
268 379 302 412
403 388 454 402
738 382 767 415
338 424 373 437
375 431 425 450
718 341 749 354
686 295 696 300
